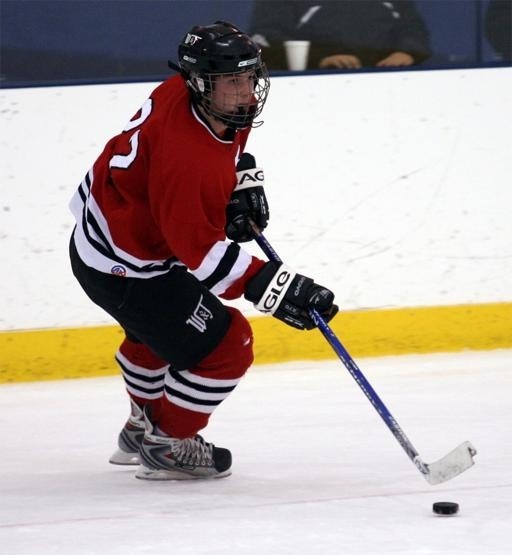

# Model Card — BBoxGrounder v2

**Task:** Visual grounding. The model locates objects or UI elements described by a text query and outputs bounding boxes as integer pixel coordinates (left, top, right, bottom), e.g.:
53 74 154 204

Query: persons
244 0 432 76
66 19 341 482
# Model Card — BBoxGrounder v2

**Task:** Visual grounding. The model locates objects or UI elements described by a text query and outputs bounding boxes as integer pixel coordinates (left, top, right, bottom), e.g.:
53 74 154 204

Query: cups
282 39 312 72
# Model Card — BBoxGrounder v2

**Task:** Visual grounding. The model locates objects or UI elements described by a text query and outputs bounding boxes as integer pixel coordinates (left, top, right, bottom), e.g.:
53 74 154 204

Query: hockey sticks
246 218 477 483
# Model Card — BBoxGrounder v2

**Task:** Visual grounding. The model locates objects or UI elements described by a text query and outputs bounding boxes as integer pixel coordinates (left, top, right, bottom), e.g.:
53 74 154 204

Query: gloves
245 257 340 330
226 153 270 240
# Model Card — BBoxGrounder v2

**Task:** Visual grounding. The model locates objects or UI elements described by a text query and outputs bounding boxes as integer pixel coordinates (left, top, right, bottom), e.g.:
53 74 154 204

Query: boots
118 388 144 456
136 407 231 476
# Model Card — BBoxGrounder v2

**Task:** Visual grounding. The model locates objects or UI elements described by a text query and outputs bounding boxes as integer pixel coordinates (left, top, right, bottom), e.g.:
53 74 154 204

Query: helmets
179 18 271 130
